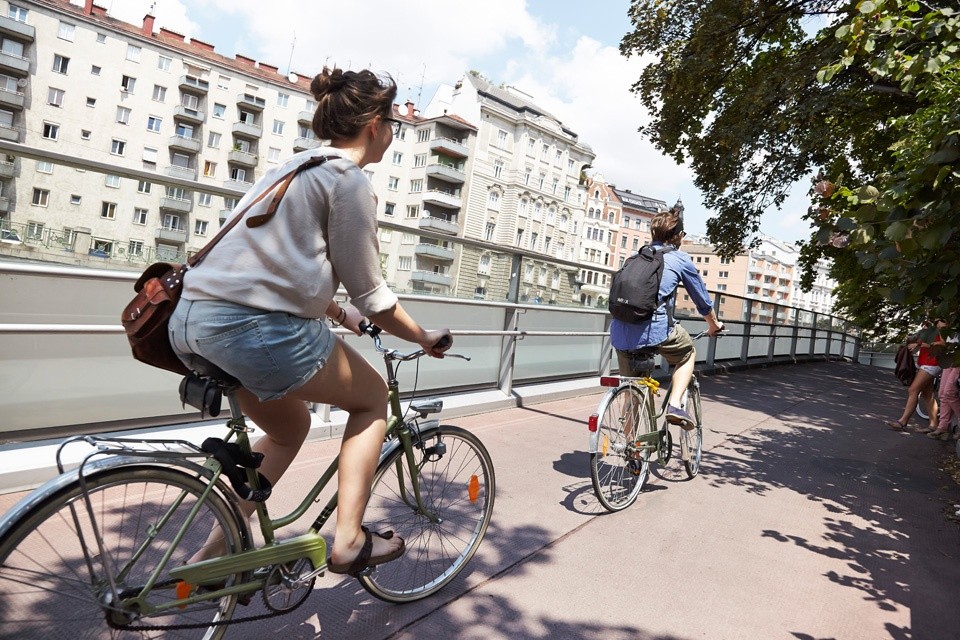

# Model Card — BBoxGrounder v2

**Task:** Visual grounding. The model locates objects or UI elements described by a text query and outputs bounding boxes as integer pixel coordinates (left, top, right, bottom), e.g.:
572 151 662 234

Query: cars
89 249 108 258
1 230 23 245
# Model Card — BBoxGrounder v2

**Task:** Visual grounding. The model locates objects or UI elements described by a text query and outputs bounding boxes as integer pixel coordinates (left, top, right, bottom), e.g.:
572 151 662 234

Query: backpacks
609 245 676 324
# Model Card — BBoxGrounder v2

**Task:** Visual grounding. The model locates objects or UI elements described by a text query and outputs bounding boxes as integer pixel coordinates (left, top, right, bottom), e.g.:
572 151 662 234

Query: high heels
953 431 960 439
927 430 948 440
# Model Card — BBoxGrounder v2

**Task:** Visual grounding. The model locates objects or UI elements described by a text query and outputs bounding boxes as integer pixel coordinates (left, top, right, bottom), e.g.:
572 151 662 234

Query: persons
168 65 453 608
886 298 960 441
609 208 725 476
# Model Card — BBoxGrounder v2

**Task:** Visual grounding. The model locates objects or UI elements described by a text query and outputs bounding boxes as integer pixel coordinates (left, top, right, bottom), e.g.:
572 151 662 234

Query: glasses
384 118 402 135
683 231 686 237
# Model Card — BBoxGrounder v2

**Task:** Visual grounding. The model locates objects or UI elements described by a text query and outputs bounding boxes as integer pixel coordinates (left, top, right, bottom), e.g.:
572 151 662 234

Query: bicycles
915 374 942 420
588 324 731 512
0 335 497 640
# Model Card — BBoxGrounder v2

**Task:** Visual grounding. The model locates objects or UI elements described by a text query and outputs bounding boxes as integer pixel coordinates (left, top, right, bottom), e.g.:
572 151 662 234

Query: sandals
889 422 907 430
917 427 935 433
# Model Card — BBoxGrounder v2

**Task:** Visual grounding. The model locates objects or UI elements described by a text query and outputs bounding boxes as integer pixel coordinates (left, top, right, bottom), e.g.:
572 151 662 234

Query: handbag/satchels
894 345 917 387
121 262 195 376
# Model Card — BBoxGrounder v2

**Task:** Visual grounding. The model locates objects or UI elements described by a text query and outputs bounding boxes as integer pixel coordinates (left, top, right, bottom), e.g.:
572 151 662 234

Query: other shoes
665 403 694 430
626 462 641 470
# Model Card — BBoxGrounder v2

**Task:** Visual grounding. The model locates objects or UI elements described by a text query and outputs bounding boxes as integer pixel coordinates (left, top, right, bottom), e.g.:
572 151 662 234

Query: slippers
176 560 251 605
327 525 406 573
